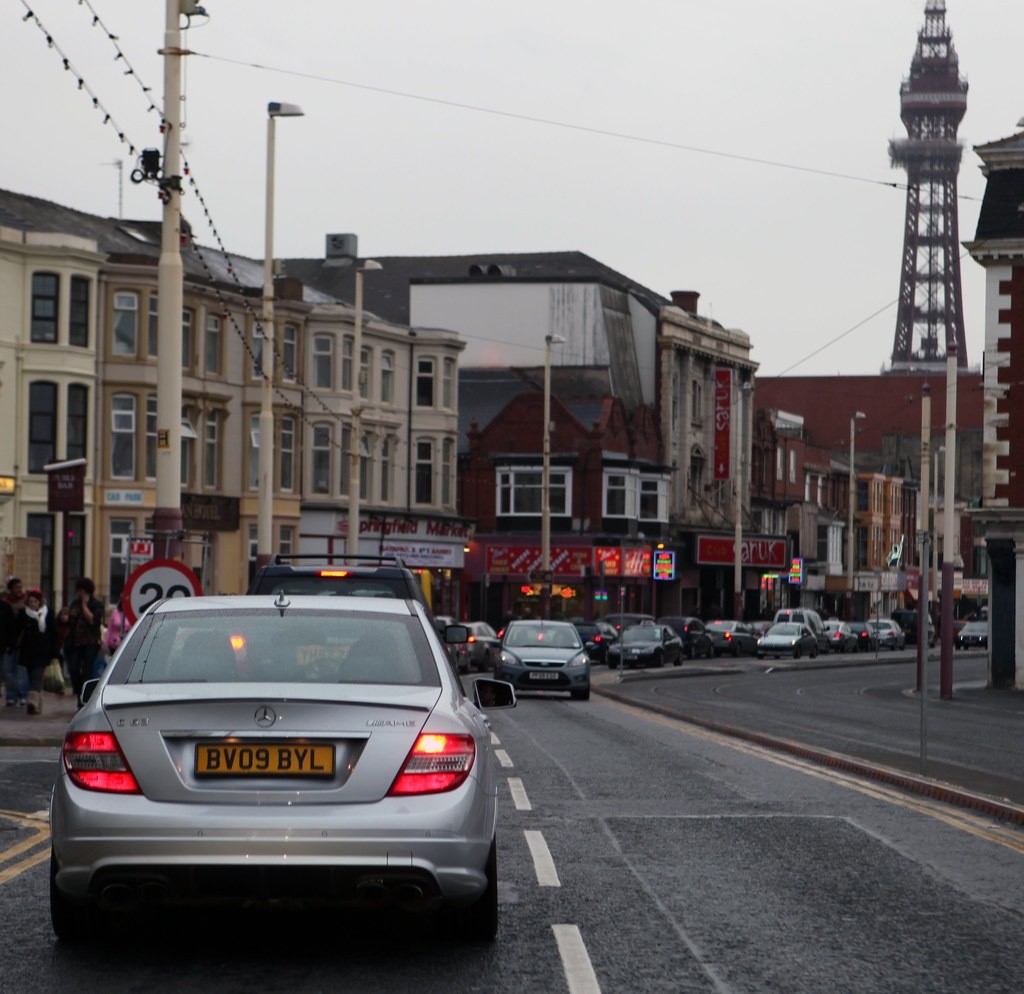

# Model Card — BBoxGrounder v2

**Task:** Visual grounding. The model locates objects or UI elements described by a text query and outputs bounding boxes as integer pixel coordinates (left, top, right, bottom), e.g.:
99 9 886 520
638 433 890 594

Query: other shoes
7 690 42 715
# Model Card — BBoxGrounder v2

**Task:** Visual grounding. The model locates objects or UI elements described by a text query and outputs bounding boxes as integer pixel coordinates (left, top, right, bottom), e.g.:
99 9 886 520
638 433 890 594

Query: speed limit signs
122 555 202 626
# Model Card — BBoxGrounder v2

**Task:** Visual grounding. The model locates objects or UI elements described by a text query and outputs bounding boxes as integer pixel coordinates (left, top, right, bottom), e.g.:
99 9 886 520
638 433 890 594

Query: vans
891 609 936 650
773 608 825 654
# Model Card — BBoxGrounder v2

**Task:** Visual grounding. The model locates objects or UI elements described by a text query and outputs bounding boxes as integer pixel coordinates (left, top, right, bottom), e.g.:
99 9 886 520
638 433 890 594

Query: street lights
348 257 383 568
257 99 303 573
736 380 752 619
540 334 565 620
848 410 866 618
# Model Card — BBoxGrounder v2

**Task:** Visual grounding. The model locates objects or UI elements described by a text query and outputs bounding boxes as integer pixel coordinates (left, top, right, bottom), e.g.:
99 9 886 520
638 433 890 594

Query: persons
66 579 103 707
12 591 58 714
107 596 129 655
1 579 23 702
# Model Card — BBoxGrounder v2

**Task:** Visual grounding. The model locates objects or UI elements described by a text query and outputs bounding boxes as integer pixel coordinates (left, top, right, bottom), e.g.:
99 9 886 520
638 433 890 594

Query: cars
758 622 819 660
574 622 617 664
867 620 905 651
449 620 499 674
657 617 715 660
705 620 758 654
607 620 684 670
486 619 596 700
955 622 988 650
823 617 858 652
848 621 878 653
51 594 518 951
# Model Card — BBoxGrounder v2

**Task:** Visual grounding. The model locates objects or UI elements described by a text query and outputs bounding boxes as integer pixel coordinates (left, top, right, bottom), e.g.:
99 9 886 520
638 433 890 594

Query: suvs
252 551 439 634
601 613 655 631
